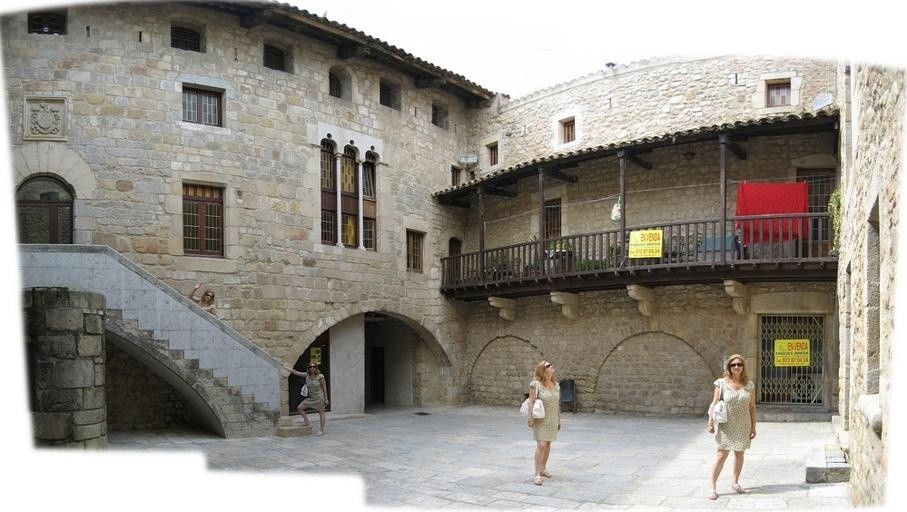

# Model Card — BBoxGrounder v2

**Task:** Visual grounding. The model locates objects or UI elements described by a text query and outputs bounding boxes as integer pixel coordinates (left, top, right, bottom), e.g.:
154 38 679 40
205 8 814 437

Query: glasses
543 364 552 370
729 363 744 368
205 293 213 298
309 365 317 368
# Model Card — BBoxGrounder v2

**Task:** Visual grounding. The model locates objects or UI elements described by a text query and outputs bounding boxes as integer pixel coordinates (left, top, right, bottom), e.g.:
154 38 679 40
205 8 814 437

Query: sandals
534 476 544 485
731 482 745 494
542 469 553 478
317 430 326 436
709 487 717 500
301 420 309 426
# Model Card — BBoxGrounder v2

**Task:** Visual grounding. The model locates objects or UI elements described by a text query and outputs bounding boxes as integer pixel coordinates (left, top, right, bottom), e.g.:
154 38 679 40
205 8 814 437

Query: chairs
657 231 739 262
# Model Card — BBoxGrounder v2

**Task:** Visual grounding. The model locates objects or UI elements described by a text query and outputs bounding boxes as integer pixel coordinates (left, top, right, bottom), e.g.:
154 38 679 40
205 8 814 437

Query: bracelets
708 422 712 425
324 398 328 401
527 416 532 421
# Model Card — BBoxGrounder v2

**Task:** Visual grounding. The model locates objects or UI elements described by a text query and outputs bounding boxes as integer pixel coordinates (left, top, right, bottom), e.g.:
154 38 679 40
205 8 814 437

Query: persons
185 281 219 317
525 359 562 487
705 352 758 500
283 360 331 436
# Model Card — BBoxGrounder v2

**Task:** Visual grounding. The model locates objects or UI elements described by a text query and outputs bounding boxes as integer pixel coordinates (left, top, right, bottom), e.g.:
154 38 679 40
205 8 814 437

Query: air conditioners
789 372 823 401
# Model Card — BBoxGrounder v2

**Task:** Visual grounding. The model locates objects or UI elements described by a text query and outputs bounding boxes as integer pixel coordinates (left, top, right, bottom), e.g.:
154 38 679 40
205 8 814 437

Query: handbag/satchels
519 380 546 419
707 379 728 424
300 384 309 397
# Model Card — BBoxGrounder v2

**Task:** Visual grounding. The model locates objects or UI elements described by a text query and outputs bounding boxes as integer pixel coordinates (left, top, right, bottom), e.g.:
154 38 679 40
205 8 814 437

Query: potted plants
485 233 629 281
